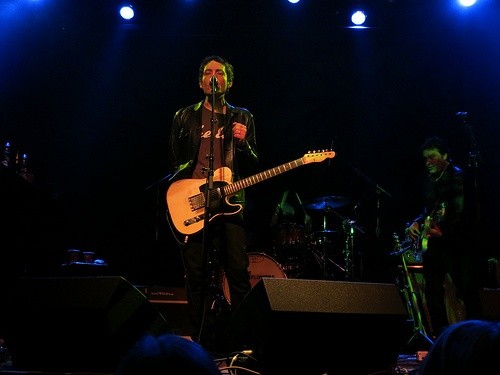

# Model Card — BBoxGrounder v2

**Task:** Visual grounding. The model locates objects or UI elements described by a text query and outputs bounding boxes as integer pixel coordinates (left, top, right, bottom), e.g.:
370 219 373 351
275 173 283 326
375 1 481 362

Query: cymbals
305 196 350 209
317 230 338 232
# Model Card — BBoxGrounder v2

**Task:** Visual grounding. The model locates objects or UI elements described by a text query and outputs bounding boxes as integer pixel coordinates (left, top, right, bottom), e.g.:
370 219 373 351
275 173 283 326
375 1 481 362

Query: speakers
0 276 172 374
226 277 408 375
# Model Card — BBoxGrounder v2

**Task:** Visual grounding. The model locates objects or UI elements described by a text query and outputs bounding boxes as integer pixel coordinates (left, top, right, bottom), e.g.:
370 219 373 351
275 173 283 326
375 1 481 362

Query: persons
404 138 484 325
173 57 257 316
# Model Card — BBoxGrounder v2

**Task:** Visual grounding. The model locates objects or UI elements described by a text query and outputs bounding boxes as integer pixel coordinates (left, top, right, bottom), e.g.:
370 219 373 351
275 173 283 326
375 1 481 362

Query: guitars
414 202 446 262
164 148 335 237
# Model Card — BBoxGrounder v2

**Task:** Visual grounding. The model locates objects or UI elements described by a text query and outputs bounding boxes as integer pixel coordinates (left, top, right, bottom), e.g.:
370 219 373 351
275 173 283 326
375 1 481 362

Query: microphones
328 141 334 168
209 76 218 86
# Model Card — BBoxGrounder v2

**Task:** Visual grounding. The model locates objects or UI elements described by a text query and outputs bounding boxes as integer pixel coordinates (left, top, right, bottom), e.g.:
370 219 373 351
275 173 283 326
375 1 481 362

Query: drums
270 223 304 248
221 253 287 306
